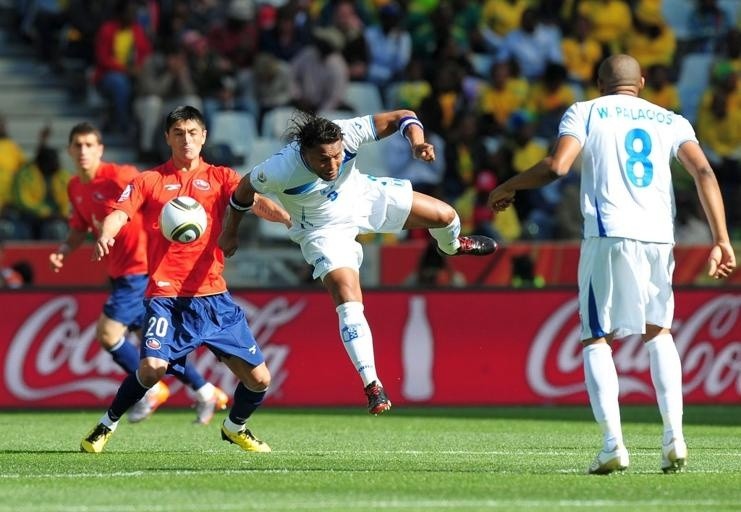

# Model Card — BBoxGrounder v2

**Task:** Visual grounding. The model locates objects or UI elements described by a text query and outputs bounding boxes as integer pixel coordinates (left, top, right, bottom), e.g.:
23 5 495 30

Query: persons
78 104 292 455
1 0 741 238
219 109 497 416
48 122 230 425
491 52 738 477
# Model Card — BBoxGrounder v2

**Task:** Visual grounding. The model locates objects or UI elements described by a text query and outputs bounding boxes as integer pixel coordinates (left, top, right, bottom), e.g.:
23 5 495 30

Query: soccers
160 196 209 243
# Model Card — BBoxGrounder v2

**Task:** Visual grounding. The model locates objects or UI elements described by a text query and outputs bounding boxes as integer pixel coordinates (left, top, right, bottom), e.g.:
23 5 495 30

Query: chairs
207 81 413 241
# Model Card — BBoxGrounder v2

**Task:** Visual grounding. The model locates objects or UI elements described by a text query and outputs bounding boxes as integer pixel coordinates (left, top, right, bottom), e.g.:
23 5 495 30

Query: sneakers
128 381 169 422
364 381 392 414
587 444 628 474
221 419 271 453
660 440 686 474
436 236 496 256
192 388 228 424
81 421 112 453
